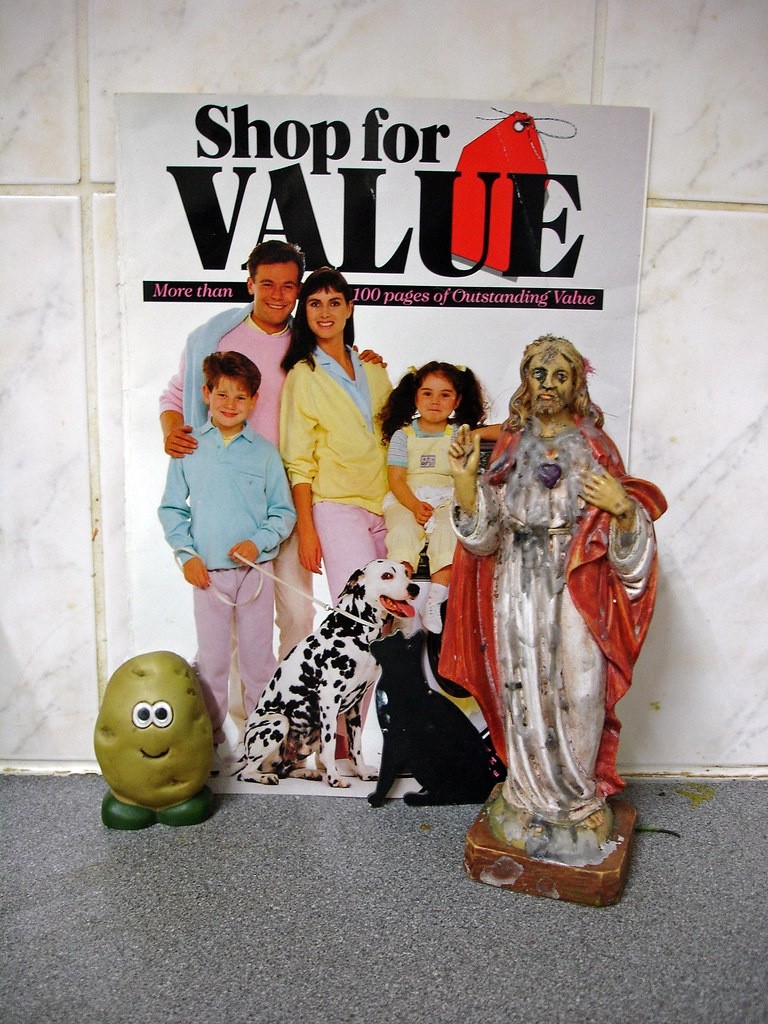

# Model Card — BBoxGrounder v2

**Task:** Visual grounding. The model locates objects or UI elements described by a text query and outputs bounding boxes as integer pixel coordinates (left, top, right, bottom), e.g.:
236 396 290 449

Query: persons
375 360 491 634
437 332 667 830
157 239 398 777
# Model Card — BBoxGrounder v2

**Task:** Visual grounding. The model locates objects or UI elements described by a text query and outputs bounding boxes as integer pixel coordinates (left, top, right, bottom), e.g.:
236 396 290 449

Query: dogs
210 558 420 789
367 629 508 807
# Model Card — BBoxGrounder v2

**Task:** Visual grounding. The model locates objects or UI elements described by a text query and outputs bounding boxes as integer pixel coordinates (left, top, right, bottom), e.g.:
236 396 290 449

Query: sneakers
419 595 443 634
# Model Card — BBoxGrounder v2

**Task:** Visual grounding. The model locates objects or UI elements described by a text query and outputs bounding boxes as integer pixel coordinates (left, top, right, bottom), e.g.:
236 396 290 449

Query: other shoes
316 754 358 776
208 752 222 779
293 758 307 768
